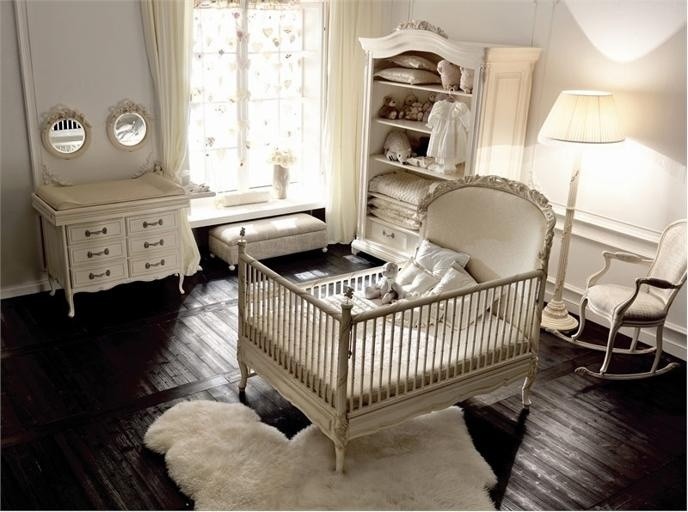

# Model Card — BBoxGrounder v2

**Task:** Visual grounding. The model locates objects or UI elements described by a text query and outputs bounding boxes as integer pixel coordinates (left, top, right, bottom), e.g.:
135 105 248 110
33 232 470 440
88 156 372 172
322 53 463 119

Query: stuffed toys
365 262 398 304
375 60 472 164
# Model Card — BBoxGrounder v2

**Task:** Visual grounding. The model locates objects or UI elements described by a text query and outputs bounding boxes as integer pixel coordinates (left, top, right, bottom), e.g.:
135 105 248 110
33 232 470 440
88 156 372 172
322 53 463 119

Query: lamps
541 89 626 332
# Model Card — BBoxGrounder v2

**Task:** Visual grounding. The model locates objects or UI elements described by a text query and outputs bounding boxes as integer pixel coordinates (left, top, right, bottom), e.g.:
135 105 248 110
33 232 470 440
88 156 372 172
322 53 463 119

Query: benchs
209 212 328 275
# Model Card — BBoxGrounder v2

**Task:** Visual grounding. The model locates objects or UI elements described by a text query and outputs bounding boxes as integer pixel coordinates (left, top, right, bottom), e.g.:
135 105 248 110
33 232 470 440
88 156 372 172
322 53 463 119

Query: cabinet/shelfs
31 172 192 319
351 17 541 267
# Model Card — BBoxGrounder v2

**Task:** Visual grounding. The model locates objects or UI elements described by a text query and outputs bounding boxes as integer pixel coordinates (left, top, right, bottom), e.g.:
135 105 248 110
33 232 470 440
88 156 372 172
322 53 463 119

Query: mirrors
38 98 153 160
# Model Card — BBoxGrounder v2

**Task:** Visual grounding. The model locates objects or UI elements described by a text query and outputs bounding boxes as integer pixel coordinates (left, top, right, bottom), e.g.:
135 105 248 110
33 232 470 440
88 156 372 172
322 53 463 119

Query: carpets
142 399 500 511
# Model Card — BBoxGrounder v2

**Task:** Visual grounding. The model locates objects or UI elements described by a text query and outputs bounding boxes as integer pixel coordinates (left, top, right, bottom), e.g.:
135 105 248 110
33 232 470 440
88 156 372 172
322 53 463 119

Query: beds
238 178 557 474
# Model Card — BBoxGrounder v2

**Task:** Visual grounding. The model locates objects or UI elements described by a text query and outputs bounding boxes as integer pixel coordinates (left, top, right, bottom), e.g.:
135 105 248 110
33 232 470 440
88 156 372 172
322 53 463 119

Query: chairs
546 219 687 382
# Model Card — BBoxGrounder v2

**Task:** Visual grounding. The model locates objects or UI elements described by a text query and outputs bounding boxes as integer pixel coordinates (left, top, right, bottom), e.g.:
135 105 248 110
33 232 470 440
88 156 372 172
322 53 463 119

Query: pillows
373 50 440 86
396 239 504 329
